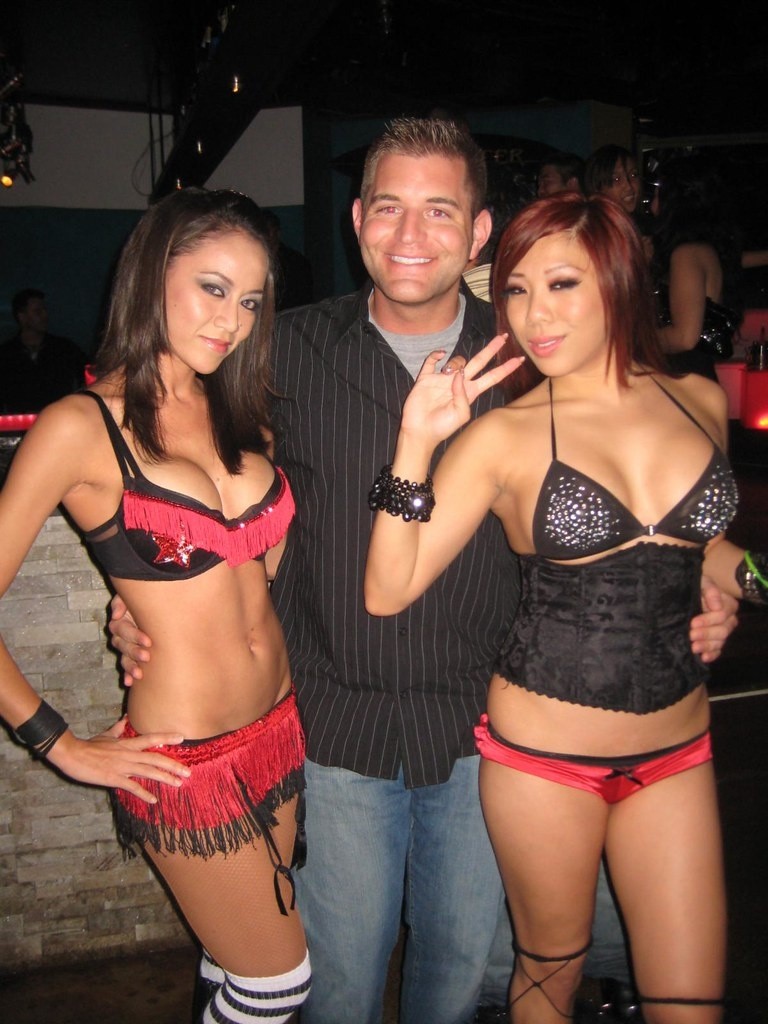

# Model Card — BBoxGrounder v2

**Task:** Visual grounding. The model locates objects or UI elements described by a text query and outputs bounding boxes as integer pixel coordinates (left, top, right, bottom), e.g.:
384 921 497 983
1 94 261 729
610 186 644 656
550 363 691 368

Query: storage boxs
714 358 747 419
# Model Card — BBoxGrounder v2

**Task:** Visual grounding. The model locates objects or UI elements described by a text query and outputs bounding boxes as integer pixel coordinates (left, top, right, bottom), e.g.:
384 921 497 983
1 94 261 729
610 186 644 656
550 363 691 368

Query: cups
752 340 768 368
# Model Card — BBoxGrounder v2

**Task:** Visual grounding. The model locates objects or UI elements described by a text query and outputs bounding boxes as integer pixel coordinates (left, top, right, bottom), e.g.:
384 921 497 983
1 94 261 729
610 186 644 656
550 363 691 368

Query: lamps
0 160 19 188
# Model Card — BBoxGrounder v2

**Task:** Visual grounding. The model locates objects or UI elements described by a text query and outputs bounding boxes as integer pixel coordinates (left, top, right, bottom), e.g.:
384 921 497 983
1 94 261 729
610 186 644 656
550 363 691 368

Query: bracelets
735 551 768 600
11 699 69 759
367 464 437 522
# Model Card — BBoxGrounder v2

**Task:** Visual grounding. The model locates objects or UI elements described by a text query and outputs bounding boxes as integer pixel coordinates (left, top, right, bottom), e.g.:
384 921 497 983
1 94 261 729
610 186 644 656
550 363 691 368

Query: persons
1 115 768 1024
